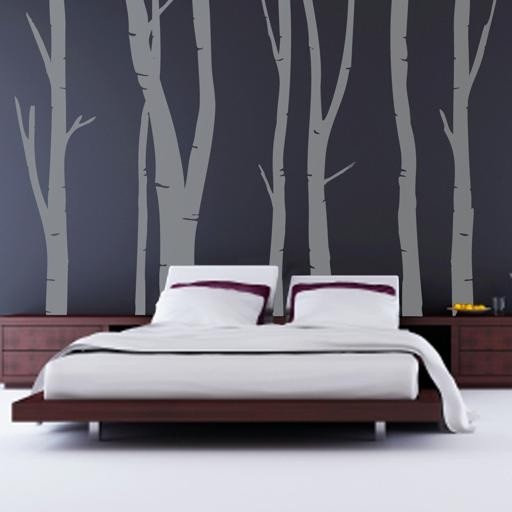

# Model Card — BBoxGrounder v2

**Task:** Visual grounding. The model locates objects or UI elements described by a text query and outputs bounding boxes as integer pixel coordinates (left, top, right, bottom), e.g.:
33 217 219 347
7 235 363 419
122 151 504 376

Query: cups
492 296 506 317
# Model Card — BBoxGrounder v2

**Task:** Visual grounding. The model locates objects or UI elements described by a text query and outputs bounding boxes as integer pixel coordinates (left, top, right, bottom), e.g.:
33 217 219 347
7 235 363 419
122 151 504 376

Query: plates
447 307 491 315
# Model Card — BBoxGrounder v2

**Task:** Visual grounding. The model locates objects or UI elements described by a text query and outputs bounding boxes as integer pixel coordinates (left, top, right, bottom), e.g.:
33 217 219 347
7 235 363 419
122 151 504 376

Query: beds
13 264 443 442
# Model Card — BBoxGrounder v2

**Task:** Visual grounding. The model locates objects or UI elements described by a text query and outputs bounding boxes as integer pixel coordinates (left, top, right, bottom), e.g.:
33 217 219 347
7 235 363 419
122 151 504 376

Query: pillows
149 279 270 327
280 282 399 329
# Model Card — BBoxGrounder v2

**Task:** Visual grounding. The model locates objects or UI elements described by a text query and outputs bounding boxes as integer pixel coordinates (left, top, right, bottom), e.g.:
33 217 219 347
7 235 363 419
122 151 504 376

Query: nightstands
451 316 512 388
0 316 110 388
